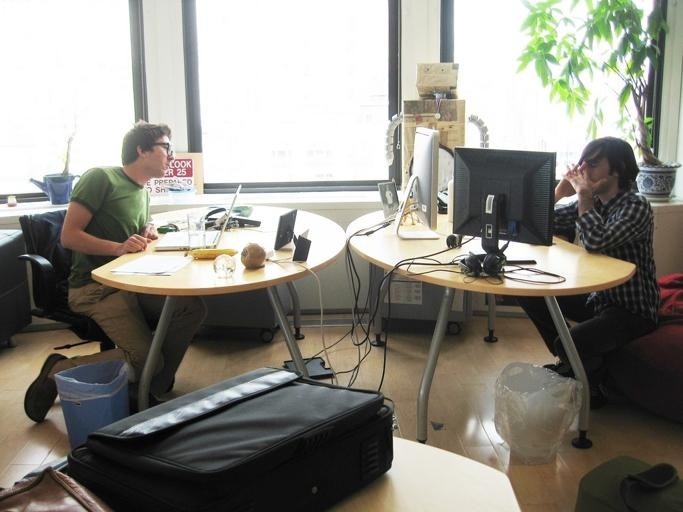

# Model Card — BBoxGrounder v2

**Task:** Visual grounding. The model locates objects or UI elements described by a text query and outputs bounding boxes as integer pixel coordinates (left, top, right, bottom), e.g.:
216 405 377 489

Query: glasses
153 142 172 155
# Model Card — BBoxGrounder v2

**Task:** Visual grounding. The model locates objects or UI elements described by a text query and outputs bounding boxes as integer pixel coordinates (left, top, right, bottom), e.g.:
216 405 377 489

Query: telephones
437 191 448 213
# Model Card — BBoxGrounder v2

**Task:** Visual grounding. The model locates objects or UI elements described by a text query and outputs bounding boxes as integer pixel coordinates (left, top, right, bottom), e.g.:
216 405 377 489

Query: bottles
6 195 18 208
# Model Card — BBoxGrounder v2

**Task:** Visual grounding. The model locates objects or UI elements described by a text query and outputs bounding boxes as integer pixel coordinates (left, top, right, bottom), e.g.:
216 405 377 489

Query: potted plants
518 0 682 201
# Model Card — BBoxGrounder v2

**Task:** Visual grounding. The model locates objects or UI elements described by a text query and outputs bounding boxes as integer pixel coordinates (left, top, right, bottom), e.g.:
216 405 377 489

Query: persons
25 122 208 424
513 136 661 406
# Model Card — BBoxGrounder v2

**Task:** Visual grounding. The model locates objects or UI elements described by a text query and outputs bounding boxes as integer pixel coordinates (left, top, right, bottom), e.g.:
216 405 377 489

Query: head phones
461 252 508 277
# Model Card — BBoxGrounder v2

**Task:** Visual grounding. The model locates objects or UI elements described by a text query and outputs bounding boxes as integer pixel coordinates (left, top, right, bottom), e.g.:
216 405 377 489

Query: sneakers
24 353 68 424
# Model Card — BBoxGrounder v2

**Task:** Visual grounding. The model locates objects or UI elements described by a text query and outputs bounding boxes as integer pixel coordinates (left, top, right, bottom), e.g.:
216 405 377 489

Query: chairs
18 208 117 352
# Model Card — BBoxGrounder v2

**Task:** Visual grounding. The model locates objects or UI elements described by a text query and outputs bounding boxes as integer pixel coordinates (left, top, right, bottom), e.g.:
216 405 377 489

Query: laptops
155 184 242 249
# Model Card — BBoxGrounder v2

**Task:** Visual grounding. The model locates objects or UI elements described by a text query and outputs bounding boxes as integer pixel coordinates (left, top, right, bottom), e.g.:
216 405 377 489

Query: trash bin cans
495 362 576 459
54 359 130 451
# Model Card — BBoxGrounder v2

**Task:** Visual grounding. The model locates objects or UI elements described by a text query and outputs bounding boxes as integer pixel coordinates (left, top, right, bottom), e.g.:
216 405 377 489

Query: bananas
191 248 238 259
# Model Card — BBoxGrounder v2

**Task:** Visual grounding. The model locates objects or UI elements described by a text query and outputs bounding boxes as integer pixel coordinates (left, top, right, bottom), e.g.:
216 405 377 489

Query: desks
347 210 637 448
89 204 345 412
333 436 522 512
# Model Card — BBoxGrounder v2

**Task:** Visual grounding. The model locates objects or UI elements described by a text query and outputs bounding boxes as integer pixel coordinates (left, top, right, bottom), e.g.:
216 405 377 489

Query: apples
241 243 266 268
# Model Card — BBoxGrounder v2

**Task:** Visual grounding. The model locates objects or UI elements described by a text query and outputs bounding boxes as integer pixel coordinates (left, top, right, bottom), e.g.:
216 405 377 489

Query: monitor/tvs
453 145 555 262
397 127 441 241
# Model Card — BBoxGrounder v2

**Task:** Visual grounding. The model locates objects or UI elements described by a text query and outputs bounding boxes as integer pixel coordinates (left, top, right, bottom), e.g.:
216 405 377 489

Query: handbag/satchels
68 365 395 512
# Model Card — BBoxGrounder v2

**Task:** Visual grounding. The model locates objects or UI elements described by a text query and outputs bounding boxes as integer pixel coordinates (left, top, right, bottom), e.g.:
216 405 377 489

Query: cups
187 211 207 252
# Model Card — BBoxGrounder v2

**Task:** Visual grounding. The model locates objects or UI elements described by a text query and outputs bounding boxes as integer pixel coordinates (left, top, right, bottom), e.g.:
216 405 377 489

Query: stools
598 272 682 423
0 229 33 348
